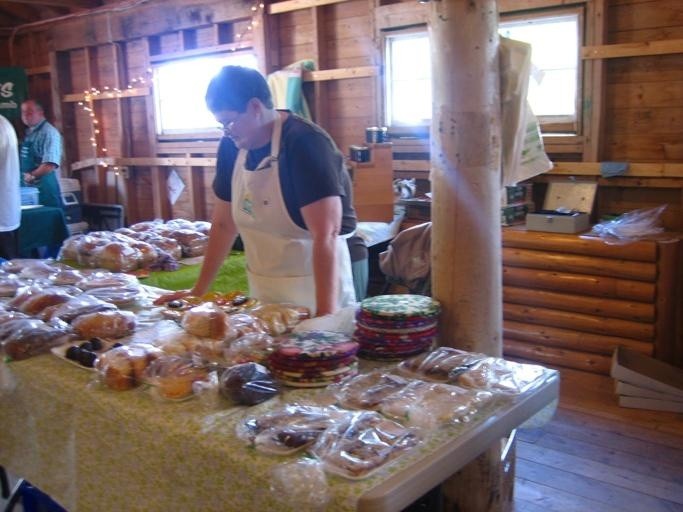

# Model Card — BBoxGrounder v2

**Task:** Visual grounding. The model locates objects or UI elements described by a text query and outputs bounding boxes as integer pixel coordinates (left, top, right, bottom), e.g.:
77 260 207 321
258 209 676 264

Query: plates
51 339 114 371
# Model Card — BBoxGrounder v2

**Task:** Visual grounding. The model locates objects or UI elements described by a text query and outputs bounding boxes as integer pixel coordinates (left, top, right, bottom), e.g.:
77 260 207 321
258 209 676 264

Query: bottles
349 126 388 163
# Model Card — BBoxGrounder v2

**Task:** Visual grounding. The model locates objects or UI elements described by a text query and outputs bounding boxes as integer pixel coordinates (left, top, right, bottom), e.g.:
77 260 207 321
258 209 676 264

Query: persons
151 64 357 318
0 113 22 258
18 101 69 258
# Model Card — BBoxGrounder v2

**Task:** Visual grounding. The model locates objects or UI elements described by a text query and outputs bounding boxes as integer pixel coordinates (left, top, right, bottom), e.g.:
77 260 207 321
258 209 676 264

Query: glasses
218 113 240 133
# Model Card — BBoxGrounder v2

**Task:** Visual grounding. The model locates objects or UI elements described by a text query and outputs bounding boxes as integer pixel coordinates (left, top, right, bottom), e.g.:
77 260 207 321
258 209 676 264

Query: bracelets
29 171 36 183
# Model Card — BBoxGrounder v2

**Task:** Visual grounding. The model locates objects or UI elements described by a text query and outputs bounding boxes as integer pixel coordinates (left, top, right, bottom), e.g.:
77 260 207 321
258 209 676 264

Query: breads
0 219 546 476
